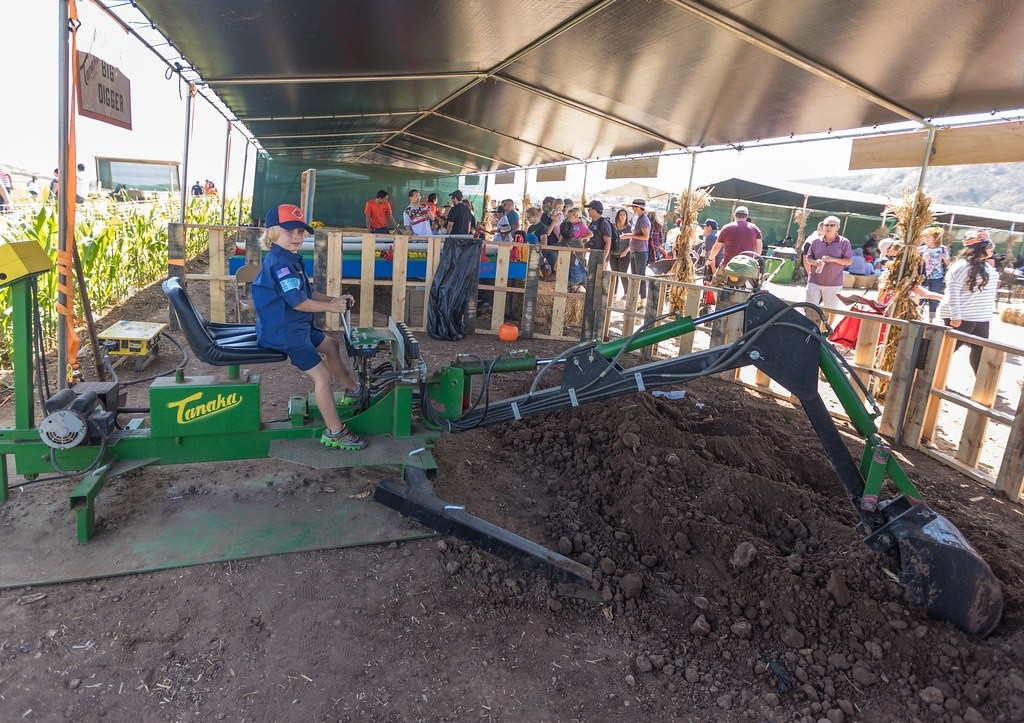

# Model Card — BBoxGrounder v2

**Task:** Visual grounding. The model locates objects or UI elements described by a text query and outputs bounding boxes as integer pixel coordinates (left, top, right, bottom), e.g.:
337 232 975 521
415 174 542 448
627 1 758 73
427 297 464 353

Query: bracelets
708 257 715 262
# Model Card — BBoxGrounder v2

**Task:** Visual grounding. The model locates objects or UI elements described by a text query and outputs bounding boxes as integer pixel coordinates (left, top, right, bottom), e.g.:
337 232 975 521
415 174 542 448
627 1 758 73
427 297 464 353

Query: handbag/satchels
942 246 949 276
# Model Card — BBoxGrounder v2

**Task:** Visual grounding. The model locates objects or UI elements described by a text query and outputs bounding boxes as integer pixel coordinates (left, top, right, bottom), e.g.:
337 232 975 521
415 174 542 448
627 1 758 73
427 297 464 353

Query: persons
360 187 1024 381
191 179 218 195
0 164 90 209
253 203 373 450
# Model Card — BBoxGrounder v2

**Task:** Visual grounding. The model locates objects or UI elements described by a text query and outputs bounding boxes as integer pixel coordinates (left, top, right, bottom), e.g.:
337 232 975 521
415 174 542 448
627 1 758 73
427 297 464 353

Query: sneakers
341 383 378 403
321 423 367 450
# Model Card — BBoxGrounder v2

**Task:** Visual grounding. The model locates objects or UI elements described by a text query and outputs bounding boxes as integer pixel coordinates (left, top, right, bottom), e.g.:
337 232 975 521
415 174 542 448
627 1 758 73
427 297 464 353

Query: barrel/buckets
768 252 797 283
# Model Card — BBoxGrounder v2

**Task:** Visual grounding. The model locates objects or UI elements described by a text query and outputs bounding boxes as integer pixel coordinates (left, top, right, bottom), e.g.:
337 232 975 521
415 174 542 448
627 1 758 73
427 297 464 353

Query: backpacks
598 217 620 251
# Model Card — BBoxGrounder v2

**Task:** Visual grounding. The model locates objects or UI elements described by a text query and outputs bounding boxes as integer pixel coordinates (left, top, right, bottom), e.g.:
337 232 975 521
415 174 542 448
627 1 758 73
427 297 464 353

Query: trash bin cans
767 245 797 283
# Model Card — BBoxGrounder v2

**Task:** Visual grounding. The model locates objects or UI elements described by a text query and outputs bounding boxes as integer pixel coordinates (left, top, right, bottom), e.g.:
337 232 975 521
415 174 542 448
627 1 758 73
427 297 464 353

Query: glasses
981 243 995 248
824 223 839 226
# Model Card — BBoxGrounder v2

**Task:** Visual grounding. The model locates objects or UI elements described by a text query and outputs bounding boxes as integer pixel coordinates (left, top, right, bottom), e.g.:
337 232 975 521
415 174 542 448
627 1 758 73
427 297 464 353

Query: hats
449 190 463 198
627 198 646 210
962 228 990 245
878 238 893 258
920 224 945 237
500 223 512 232
265 203 314 234
701 218 717 229
584 200 603 211
491 205 505 212
735 206 748 214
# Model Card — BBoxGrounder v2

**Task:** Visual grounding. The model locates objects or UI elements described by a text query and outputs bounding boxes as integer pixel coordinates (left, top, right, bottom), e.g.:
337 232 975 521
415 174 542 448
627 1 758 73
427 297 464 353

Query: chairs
162 276 287 365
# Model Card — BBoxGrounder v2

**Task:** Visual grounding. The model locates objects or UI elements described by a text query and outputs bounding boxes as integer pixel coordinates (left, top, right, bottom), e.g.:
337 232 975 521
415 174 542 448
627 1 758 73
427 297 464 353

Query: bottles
816 256 825 274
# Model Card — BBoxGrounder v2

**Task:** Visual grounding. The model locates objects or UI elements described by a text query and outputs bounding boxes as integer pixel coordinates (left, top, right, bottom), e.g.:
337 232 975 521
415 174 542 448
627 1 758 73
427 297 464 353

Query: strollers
724 251 785 293
553 241 587 293
820 286 894 382
